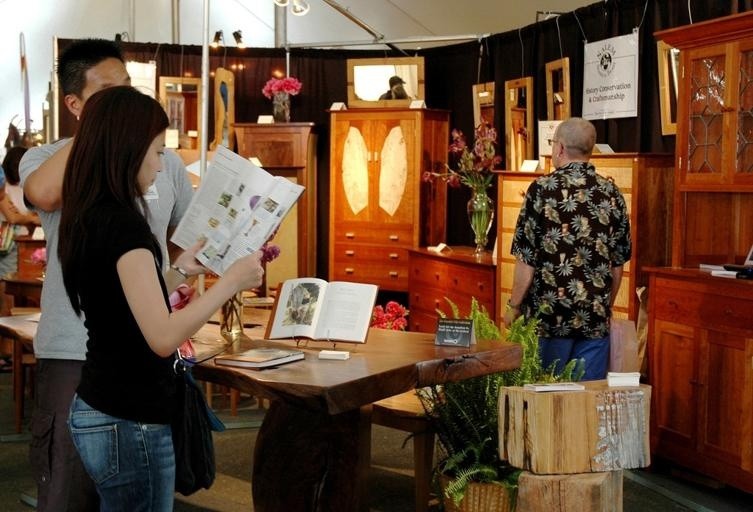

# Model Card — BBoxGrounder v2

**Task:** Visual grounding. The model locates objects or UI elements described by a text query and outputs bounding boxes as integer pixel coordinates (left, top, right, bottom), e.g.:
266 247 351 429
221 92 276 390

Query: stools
360 384 455 510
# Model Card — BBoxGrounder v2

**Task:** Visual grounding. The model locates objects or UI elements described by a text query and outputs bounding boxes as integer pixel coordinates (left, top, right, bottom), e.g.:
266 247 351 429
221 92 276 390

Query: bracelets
506 297 523 311
169 263 189 278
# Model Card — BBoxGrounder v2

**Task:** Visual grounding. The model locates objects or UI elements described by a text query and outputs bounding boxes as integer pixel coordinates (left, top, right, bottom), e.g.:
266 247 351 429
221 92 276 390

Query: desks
0 298 533 509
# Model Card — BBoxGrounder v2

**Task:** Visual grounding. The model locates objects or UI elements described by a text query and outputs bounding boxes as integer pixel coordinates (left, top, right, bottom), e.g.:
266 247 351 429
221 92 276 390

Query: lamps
209 28 222 48
231 29 246 48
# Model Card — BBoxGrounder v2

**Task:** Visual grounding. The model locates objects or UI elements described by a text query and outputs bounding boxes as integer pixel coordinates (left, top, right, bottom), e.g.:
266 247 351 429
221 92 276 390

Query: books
527 383 582 391
170 144 304 278
263 277 379 345
212 348 305 373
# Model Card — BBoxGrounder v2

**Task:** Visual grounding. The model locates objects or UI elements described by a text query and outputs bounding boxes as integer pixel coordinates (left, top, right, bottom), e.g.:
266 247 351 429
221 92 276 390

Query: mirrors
472 79 499 138
209 68 236 150
157 72 200 155
502 75 534 175
544 56 571 124
653 39 680 137
343 54 427 112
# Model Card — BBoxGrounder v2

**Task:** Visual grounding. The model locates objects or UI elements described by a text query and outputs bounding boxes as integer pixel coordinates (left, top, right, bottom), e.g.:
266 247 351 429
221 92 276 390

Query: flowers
418 115 511 244
262 229 283 263
263 77 302 124
365 298 412 334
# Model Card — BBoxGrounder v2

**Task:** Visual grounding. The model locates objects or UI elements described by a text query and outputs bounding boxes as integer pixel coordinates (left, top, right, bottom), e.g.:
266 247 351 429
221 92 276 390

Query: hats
389 76 406 88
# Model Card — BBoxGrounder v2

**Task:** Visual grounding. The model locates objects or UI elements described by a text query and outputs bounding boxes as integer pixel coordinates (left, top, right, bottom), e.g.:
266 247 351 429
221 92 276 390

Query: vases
220 290 243 339
465 184 495 252
270 93 291 122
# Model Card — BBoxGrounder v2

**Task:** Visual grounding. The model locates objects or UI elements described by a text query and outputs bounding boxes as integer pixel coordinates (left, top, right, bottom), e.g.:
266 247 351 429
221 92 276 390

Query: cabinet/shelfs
488 167 548 328
636 260 752 504
231 118 319 294
651 6 752 199
402 243 497 335
325 107 450 304
0 214 45 369
543 151 678 339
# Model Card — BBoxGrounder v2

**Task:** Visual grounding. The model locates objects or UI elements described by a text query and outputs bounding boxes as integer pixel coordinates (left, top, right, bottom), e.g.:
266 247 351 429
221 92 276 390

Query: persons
381 76 411 100
57 82 265 507
505 118 632 379
0 166 43 226
296 289 310 324
18 36 197 508
0 146 30 372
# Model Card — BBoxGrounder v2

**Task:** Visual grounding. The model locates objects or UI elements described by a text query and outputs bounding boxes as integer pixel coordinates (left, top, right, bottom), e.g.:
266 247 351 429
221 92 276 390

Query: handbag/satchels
171 359 226 496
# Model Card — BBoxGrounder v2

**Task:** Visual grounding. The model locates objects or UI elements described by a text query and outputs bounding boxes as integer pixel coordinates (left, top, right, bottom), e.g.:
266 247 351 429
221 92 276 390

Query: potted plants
419 294 588 511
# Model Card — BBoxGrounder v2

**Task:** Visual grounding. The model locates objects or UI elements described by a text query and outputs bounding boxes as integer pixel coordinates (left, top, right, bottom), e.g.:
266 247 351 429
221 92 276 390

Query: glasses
547 139 559 145
